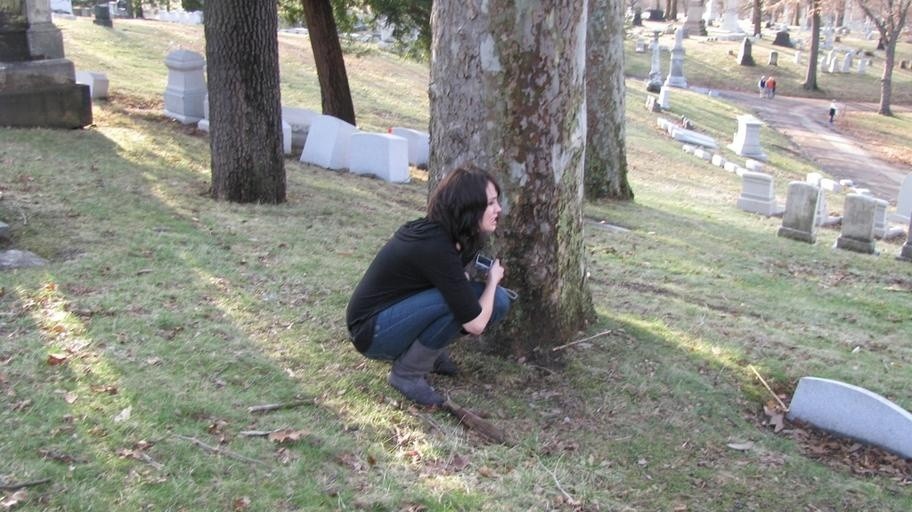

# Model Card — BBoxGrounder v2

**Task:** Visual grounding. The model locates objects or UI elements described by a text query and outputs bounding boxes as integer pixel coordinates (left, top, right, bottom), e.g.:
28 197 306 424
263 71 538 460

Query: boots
389 339 457 404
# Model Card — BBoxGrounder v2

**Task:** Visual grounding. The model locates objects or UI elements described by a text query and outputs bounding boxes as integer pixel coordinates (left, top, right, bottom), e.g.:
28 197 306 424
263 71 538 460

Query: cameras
473 254 493 271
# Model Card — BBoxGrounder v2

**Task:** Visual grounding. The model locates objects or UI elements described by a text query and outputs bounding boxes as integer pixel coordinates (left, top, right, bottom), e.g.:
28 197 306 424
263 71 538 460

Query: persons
828 99 839 123
766 77 773 100
758 75 765 98
772 78 776 96
345 165 511 409
